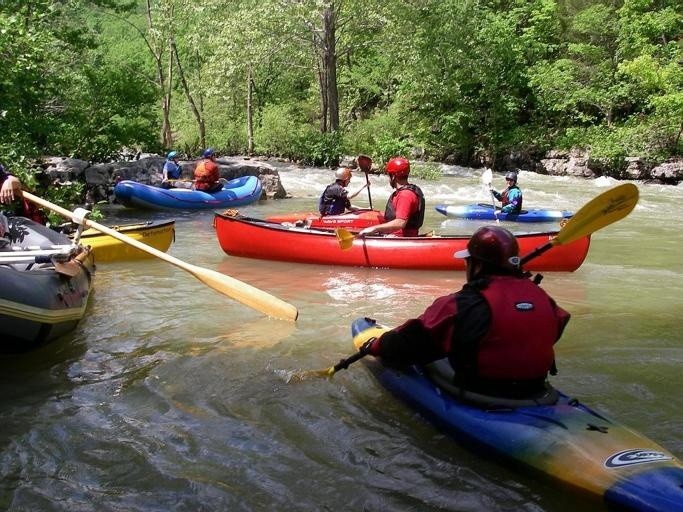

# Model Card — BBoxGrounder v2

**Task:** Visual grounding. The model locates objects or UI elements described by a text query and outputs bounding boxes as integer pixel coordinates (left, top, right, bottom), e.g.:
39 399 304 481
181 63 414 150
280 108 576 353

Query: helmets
335 167 352 181
387 158 410 176
454 225 520 270
204 148 215 159
167 151 179 161
505 171 518 185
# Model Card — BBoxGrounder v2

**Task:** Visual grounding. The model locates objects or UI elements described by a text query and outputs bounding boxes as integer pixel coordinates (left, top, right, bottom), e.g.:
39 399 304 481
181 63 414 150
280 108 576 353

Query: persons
359 158 421 237
319 168 359 216
195 149 224 193
0 163 23 240
490 172 522 215
361 228 558 402
161 151 185 190
20 180 44 225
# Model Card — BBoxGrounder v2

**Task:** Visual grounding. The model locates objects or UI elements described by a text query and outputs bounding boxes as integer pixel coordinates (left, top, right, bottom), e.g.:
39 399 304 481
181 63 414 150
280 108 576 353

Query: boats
1 216 94 343
56 220 175 261
436 203 575 220
351 317 682 511
213 210 591 271
117 176 262 206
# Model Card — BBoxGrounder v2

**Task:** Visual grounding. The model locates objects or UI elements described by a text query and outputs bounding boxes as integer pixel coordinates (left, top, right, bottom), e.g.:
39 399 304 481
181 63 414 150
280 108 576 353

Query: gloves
361 337 384 357
358 227 375 236
489 188 496 194
494 210 501 216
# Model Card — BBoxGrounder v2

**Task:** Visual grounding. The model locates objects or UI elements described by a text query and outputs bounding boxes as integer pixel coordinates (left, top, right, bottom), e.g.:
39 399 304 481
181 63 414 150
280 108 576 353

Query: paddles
13 188 298 324
167 178 229 185
357 155 372 210
334 227 433 250
480 168 500 222
291 183 638 382
